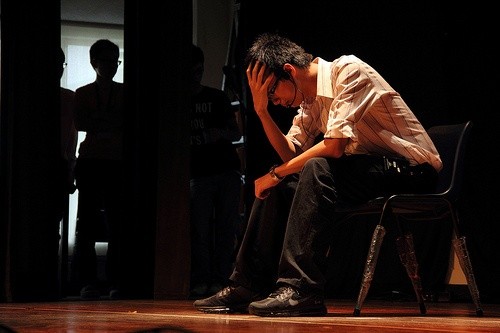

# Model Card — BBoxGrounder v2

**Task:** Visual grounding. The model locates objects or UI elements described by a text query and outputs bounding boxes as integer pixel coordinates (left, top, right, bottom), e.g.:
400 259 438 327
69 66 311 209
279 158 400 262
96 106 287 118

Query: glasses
268 72 281 100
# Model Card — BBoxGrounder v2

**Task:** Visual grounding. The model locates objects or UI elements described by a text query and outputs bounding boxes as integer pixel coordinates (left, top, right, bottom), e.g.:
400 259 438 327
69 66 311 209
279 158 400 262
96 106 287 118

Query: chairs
322 120 483 316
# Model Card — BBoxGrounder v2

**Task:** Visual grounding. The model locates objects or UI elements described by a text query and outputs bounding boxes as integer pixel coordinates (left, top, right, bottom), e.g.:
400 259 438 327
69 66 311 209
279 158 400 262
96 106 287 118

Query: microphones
282 71 296 108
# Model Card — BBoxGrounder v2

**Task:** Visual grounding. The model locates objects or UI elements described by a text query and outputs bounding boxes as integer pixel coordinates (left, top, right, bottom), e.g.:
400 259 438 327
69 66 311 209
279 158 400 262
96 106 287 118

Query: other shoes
81 286 101 298
110 288 129 299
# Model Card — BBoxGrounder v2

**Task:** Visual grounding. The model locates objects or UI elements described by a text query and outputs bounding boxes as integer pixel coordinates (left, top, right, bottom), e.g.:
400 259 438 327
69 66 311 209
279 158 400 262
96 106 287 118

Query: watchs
269 164 286 183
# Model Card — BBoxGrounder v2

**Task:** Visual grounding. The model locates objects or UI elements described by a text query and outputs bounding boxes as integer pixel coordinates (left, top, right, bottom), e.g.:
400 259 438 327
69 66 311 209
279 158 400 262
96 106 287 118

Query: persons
149 43 242 296
5 43 77 274
70 40 147 297
193 35 444 316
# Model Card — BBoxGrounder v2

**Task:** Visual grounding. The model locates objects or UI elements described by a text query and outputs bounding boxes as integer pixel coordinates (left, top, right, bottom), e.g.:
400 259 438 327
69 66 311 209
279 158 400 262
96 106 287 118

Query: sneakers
192 285 251 310
248 287 327 317
